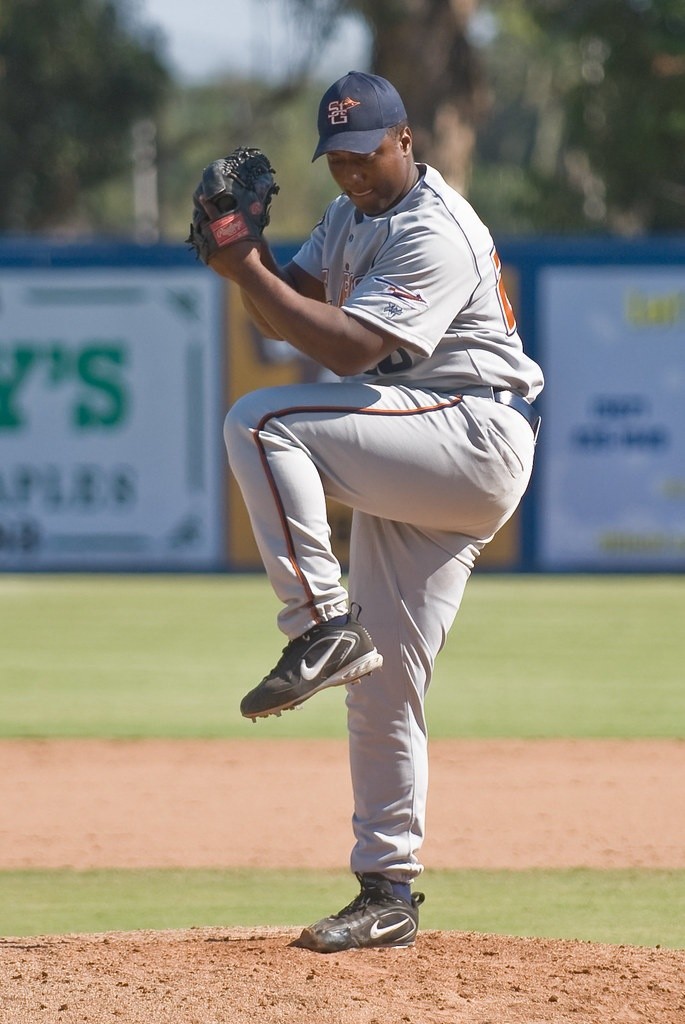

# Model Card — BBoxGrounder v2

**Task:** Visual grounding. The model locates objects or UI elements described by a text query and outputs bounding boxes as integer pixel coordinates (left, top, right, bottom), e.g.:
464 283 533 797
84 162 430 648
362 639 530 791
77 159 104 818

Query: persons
192 72 543 952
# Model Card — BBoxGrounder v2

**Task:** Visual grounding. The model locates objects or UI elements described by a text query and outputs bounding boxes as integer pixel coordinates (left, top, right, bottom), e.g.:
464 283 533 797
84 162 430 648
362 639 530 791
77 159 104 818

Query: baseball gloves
183 146 281 265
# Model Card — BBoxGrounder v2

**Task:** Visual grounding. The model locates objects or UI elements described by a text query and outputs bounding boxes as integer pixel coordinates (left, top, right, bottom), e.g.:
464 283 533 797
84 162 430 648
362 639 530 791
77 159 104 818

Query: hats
311 71 407 163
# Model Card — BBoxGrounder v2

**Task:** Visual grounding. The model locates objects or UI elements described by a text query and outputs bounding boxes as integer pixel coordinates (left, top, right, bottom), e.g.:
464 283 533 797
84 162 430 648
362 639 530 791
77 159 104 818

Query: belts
493 386 539 434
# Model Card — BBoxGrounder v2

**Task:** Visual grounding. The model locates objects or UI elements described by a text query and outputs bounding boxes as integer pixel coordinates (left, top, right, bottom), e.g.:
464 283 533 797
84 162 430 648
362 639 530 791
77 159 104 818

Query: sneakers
240 602 383 723
300 871 425 953
